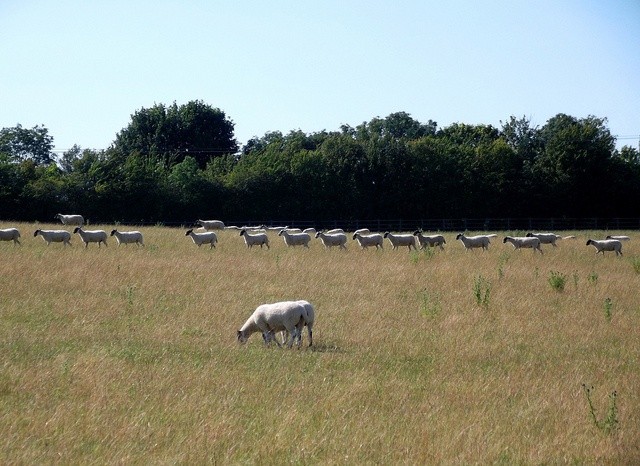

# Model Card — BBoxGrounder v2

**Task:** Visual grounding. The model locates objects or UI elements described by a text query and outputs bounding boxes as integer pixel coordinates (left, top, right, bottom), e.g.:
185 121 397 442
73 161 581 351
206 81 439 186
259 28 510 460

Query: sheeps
526 233 558 247
196 226 206 232
456 234 491 252
73 225 108 248
236 302 310 351
224 226 238 230
185 229 217 250
285 228 301 233
251 229 266 233
606 235 630 241
586 239 622 257
383 231 418 251
262 300 314 347
0 228 21 247
556 235 562 240
303 228 316 234
353 228 369 240
54 213 85 227
241 225 263 230
261 224 287 232
278 230 311 249
413 230 446 253
315 232 347 251
194 218 225 231
110 229 144 249
354 234 384 251
34 228 72 247
319 229 344 234
240 229 270 249
503 236 543 254
